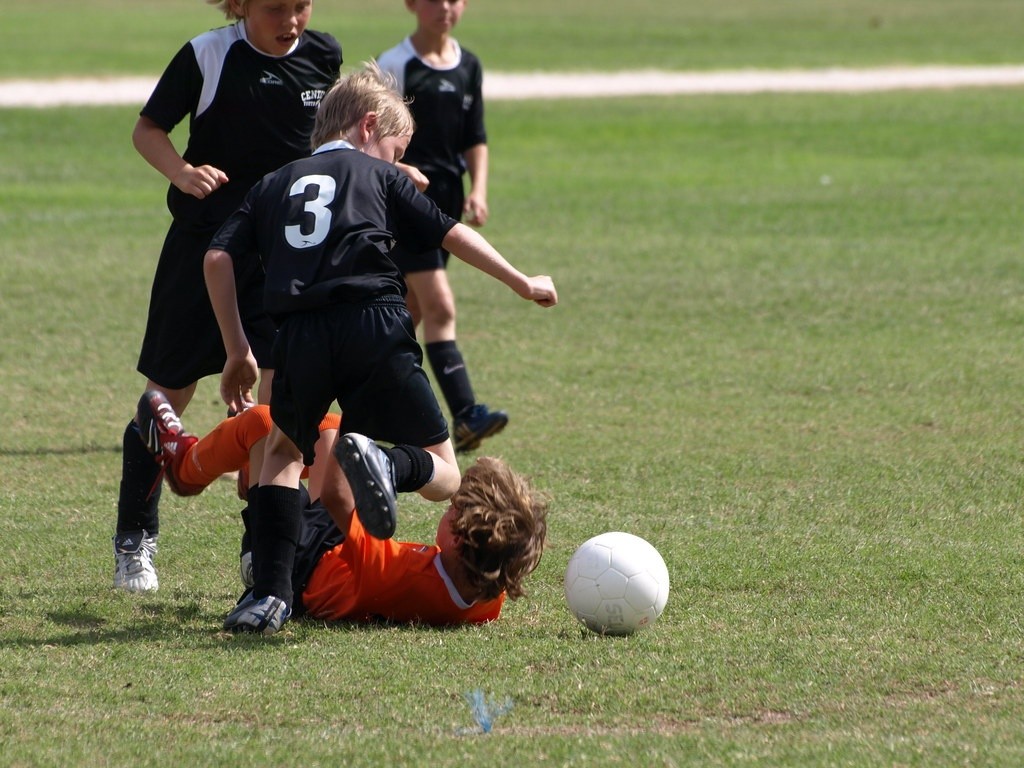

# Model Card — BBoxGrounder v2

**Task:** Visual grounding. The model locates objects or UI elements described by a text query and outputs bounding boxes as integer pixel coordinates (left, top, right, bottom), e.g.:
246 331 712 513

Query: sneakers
112 529 159 595
227 408 250 501
240 550 256 588
137 390 205 501
223 589 293 636
453 405 508 452
335 432 397 539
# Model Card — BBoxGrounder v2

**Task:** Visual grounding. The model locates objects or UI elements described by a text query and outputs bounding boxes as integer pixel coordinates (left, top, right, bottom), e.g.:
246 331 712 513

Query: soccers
564 531 671 636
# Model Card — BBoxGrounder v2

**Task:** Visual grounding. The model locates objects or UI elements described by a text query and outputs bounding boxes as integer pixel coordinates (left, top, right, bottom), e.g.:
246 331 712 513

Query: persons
373 0 509 452
137 390 549 626
203 68 558 632
114 0 343 594
